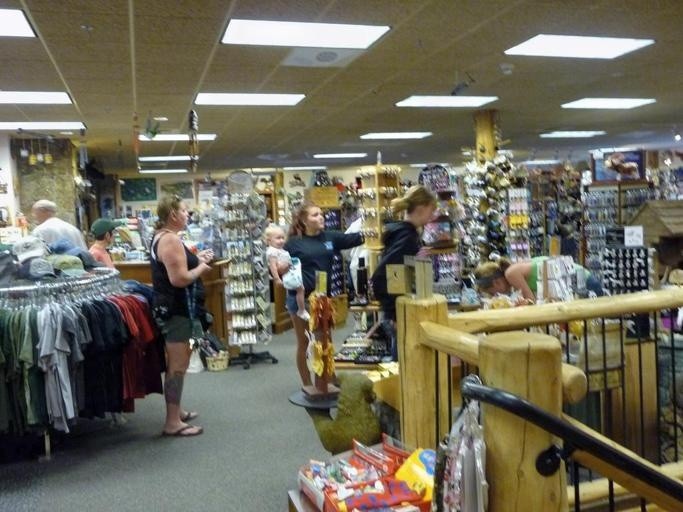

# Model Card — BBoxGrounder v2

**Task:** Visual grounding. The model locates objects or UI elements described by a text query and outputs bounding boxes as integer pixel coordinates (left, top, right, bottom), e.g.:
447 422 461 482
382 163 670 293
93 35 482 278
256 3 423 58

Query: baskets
205 356 228 371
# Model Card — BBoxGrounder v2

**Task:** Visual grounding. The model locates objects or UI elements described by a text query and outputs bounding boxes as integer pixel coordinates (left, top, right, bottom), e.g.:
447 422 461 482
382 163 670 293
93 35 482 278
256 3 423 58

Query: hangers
0 270 156 328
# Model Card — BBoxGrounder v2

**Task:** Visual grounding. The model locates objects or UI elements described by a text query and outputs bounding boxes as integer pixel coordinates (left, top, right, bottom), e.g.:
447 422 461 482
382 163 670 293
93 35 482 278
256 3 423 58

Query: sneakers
296 310 312 322
349 295 368 306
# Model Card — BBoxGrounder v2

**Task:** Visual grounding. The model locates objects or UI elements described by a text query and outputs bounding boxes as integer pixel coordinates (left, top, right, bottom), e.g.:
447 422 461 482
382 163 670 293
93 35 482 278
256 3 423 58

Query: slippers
162 425 204 436
183 411 197 420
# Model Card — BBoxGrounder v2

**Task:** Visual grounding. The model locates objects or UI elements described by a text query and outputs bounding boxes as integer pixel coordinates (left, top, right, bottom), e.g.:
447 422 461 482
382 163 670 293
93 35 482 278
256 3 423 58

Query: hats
0 235 107 277
91 218 122 238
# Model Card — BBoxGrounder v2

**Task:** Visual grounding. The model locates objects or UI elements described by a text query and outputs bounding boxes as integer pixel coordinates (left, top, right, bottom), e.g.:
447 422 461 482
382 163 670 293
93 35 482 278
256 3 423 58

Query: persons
372 185 436 361
149 194 214 435
264 204 365 386
475 256 603 301
31 200 121 268
